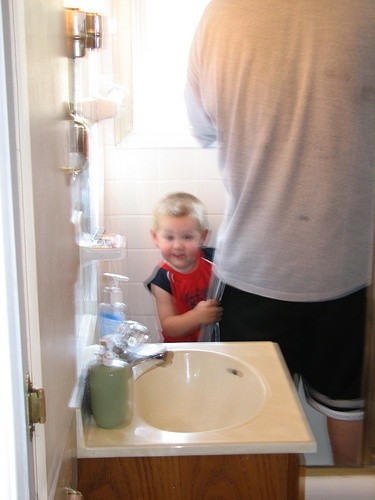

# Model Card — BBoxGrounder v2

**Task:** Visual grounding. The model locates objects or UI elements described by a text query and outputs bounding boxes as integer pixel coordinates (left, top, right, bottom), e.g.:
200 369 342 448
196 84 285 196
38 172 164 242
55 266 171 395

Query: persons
181 0 375 467
143 190 223 342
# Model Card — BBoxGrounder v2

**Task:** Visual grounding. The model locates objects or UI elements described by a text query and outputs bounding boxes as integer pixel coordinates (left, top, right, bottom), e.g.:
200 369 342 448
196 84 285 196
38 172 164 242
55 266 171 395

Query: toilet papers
202 218 267 297
